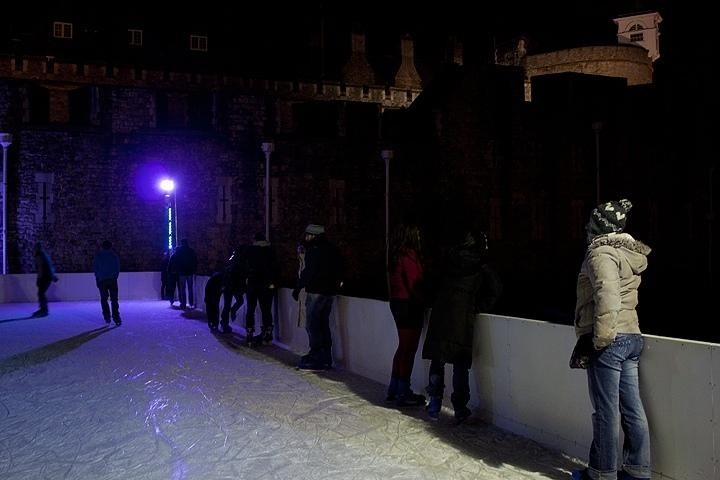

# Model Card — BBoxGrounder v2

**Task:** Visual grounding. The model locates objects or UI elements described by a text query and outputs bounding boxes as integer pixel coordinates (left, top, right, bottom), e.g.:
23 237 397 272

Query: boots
246 325 273 342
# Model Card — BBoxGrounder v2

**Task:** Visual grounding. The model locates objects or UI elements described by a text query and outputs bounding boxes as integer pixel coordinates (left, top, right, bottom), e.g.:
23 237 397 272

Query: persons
93 239 122 327
385 220 429 408
567 193 656 479
30 239 60 319
158 222 347 374
421 226 499 424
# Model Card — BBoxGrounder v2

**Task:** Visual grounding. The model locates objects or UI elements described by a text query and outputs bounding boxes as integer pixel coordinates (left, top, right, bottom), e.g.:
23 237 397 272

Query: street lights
591 119 606 207
0 132 13 273
379 146 394 273
158 176 176 256
261 140 274 243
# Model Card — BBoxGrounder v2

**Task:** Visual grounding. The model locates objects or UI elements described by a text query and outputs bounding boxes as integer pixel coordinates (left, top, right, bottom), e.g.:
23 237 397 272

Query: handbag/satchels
569 333 606 370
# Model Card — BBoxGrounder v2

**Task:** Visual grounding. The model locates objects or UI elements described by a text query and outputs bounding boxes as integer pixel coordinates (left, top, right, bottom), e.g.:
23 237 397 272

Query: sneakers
571 468 649 480
385 394 471 422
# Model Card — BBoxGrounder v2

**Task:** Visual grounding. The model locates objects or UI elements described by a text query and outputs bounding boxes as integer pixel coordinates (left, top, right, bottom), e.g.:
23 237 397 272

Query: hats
304 223 325 235
585 199 632 235
